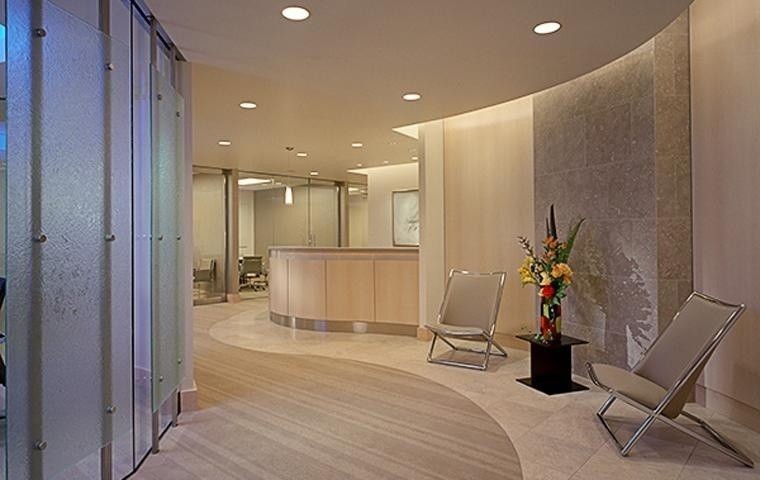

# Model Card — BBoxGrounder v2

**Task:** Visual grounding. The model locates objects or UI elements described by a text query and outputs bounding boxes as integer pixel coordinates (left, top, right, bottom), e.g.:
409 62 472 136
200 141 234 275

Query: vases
541 285 562 341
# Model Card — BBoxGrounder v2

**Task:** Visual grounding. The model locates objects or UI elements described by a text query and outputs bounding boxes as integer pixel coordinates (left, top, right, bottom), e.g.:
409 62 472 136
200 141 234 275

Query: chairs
585 292 754 468
193 256 214 296
239 256 266 292
423 268 507 371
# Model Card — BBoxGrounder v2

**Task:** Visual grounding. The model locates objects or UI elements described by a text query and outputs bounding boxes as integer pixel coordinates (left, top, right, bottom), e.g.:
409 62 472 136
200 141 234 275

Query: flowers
515 204 586 289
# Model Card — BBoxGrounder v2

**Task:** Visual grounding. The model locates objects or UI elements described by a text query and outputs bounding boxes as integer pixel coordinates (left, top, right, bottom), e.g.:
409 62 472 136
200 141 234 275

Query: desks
515 333 589 395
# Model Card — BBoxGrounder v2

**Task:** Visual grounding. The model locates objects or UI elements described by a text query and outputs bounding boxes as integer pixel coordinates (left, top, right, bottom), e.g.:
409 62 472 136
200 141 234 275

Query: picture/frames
391 189 420 247
